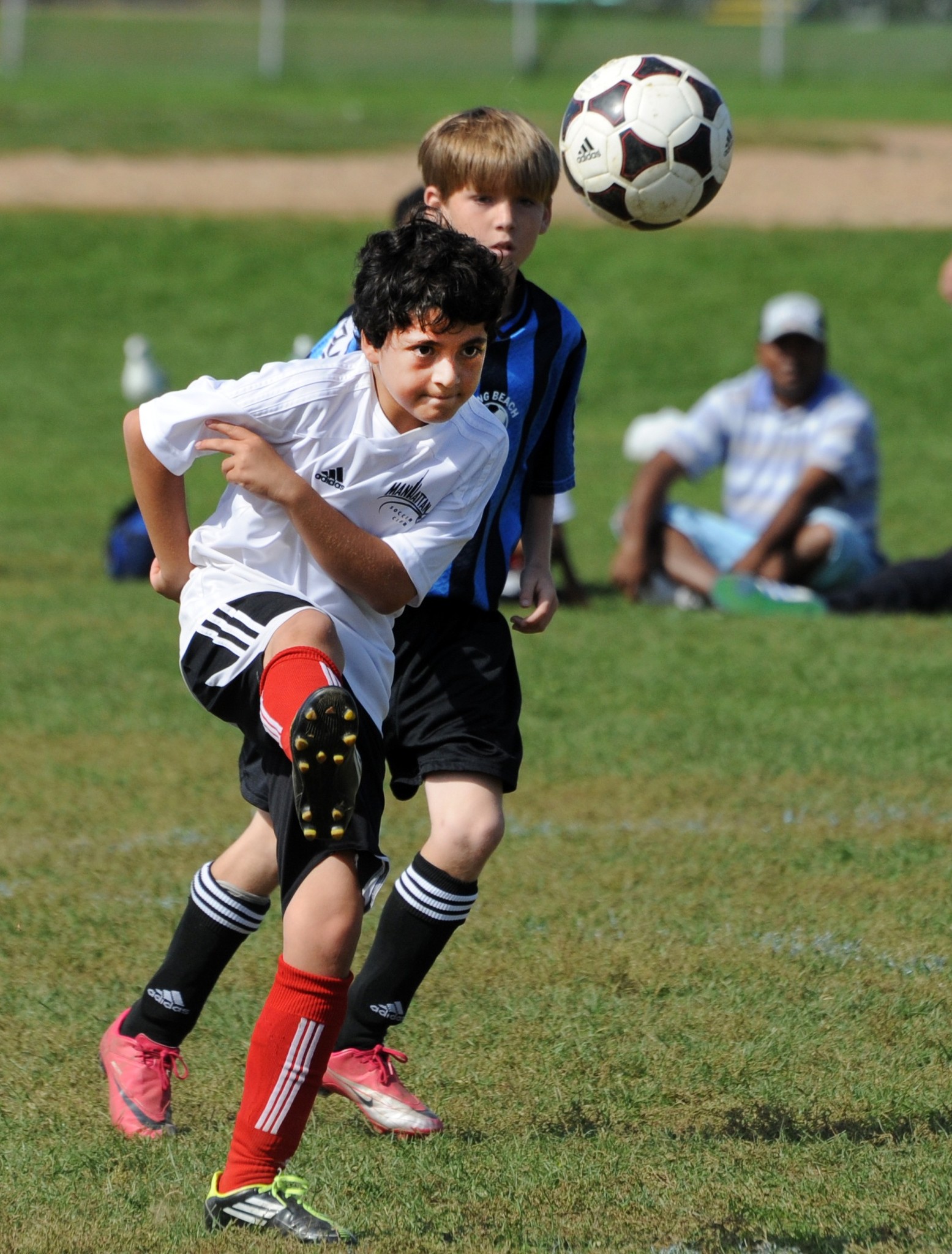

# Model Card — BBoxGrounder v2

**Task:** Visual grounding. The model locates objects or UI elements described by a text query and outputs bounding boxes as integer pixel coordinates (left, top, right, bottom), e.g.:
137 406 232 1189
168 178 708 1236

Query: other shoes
670 584 702 612
757 578 820 603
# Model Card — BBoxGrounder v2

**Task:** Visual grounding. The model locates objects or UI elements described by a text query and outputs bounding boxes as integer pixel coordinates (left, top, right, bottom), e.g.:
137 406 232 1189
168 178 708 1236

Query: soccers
559 52 737 232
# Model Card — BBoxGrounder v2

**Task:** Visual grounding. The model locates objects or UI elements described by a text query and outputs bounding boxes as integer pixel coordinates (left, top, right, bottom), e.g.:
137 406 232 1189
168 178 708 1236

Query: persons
607 285 883 627
122 198 511 1244
92 98 626 1151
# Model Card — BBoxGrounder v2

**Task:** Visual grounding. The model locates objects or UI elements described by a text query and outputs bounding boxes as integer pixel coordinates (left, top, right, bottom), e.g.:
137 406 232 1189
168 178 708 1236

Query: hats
761 291 827 344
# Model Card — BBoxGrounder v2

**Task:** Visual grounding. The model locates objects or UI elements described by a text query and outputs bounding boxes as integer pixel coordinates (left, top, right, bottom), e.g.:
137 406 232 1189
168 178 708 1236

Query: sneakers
290 686 363 846
320 1044 443 1138
206 1166 360 1248
98 1008 188 1141
